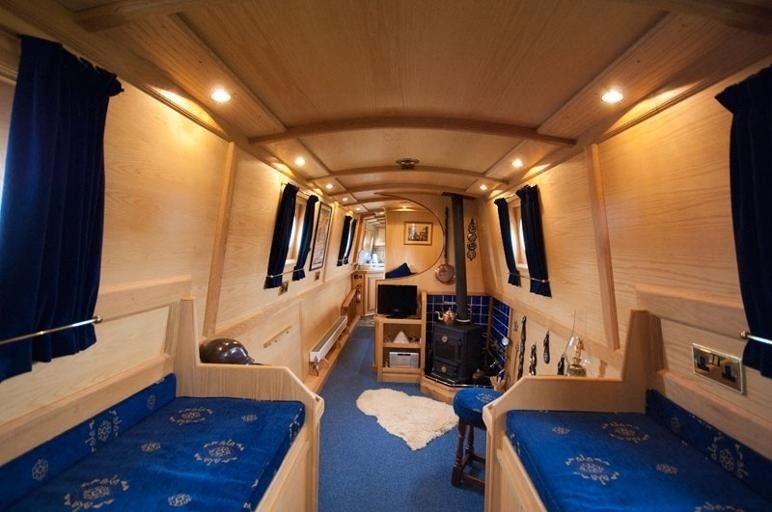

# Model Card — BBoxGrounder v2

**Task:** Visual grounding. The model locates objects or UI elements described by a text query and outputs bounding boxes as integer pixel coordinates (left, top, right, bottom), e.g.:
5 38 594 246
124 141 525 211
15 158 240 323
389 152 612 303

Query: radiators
342 289 357 325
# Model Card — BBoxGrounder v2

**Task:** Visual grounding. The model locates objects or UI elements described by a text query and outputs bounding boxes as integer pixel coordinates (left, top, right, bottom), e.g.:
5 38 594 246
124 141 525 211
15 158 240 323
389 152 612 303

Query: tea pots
435 306 458 325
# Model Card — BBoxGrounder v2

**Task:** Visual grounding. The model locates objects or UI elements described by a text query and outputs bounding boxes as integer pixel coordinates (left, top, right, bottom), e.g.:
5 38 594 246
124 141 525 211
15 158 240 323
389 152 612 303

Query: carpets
356 388 460 451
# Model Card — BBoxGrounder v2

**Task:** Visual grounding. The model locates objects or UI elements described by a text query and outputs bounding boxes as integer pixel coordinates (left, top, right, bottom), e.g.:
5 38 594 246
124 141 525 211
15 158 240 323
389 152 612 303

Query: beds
483 288 771 512
0 276 325 511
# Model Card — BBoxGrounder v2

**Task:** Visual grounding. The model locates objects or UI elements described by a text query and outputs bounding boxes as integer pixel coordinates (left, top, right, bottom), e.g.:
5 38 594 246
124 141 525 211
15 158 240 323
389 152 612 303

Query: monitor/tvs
377 284 417 319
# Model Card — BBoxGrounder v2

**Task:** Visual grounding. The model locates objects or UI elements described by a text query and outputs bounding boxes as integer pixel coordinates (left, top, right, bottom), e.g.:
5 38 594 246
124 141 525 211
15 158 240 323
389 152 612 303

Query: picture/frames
309 201 332 271
404 222 433 245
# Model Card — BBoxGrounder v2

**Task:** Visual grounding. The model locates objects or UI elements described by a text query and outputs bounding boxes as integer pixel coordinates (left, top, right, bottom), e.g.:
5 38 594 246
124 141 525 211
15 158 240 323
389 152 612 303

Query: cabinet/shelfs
351 270 384 317
373 290 427 383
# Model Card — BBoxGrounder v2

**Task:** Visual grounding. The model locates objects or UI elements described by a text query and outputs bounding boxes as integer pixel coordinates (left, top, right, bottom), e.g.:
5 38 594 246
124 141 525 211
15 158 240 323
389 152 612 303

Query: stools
452 388 504 493
